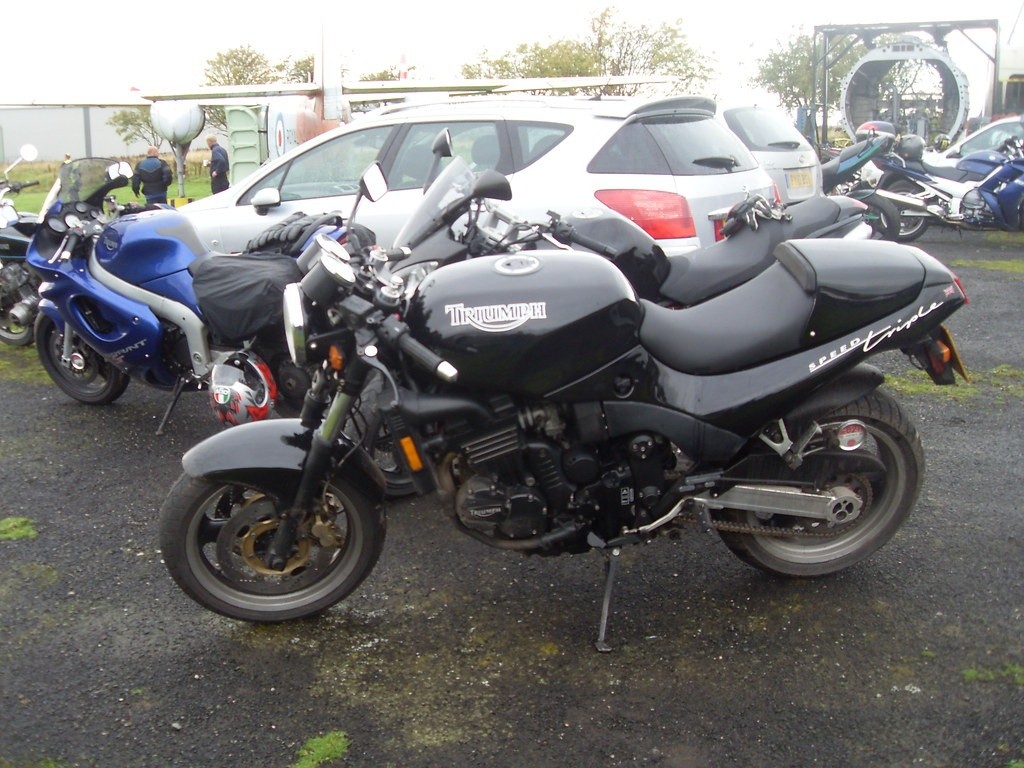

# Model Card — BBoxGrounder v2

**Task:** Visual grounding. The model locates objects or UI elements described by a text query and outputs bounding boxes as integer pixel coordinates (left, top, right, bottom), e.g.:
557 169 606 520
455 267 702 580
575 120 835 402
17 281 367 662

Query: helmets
209 348 277 430
898 134 925 162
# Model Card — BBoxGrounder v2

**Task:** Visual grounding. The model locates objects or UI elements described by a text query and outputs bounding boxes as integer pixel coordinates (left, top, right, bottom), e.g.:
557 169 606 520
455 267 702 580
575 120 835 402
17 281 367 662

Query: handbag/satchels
192 253 304 345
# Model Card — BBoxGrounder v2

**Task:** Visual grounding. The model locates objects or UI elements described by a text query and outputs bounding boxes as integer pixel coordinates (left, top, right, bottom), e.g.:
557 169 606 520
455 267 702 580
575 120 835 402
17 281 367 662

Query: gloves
719 193 793 237
245 211 335 252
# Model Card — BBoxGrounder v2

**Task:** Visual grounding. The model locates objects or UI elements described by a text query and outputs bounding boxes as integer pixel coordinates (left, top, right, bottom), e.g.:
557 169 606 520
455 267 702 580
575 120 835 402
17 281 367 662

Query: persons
60 152 81 203
132 148 172 204
203 136 229 194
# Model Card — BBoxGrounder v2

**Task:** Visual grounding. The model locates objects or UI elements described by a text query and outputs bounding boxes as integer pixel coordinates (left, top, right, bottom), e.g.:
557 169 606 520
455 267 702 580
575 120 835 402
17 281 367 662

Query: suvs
166 86 825 304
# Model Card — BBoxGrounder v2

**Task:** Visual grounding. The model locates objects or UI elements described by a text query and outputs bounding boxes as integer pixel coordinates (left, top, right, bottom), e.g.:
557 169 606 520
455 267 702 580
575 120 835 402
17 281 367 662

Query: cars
933 114 1024 159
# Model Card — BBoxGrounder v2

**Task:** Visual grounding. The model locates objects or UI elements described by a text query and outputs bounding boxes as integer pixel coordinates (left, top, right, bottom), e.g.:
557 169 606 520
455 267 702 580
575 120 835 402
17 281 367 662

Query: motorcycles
25 155 363 436
158 120 1023 655
0 143 180 348
